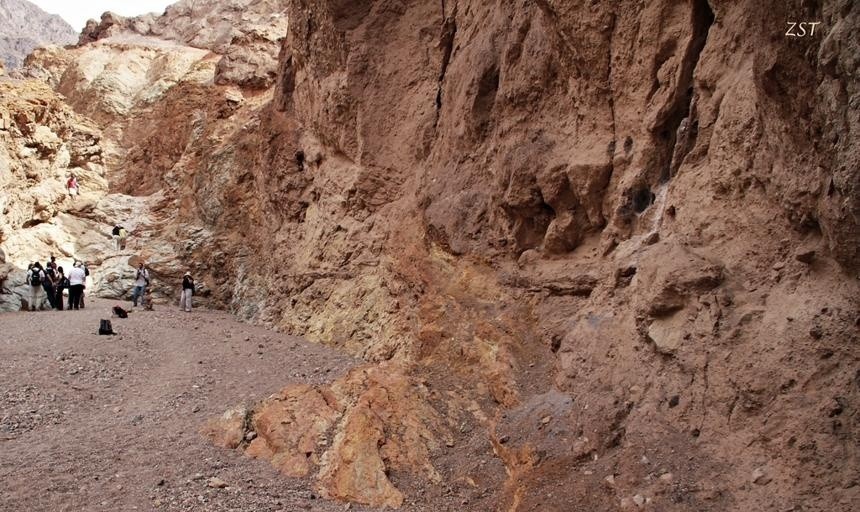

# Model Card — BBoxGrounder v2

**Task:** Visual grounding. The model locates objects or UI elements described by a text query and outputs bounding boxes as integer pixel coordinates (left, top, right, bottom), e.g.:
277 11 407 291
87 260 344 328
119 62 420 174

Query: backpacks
43 268 55 287
30 268 41 286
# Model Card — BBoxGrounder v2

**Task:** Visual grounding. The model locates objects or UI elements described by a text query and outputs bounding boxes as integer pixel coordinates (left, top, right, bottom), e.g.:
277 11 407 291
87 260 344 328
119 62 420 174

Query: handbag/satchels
56 277 70 288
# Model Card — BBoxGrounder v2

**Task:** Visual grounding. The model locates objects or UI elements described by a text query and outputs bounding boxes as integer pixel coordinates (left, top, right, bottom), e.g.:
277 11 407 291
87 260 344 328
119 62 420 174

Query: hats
182 271 192 278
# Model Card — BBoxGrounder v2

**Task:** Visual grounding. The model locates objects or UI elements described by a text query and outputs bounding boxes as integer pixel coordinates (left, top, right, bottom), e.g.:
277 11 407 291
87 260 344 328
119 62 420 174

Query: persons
26 256 92 313
118 227 128 251
176 270 197 311
132 263 149 305
67 174 80 197
111 225 123 253
